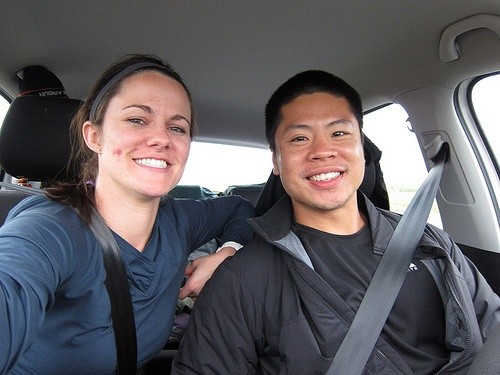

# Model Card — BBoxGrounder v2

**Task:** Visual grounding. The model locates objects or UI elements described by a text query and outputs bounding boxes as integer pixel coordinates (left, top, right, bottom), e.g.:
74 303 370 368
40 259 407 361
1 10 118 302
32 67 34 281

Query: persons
172 70 500 375
1 54 257 375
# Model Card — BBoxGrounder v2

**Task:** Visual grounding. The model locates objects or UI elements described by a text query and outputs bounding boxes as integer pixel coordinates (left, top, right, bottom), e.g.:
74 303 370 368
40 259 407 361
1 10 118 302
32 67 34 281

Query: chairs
0 95 390 227
